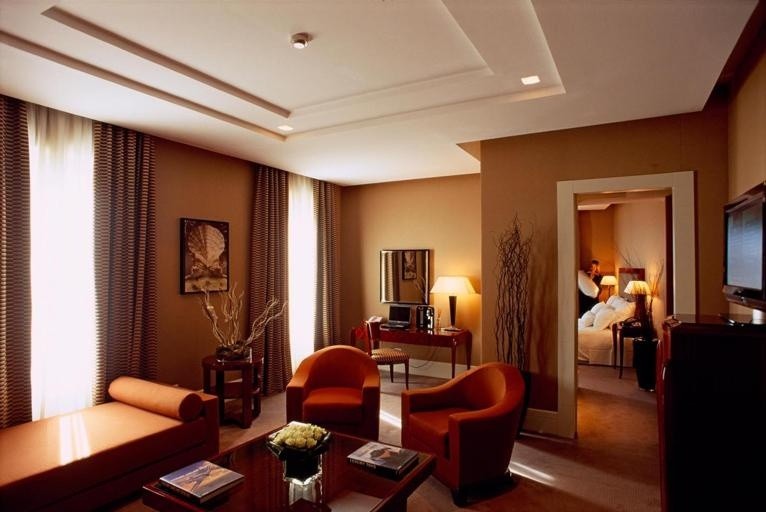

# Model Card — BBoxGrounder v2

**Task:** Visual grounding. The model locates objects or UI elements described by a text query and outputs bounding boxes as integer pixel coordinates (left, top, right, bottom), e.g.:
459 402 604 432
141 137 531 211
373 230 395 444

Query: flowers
267 421 328 455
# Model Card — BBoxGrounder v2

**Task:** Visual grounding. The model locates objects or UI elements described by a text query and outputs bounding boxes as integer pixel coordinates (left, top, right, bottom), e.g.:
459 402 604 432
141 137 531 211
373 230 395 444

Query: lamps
429 275 481 329
624 280 651 318
600 275 617 298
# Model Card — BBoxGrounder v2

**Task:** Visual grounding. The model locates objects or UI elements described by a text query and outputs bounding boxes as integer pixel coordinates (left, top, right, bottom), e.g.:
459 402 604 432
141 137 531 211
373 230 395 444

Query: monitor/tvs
381 305 411 324
719 181 766 329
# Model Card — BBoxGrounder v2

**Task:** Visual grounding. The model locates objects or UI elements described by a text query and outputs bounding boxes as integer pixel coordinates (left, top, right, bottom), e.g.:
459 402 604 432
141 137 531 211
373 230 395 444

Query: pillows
581 293 637 330
105 375 203 422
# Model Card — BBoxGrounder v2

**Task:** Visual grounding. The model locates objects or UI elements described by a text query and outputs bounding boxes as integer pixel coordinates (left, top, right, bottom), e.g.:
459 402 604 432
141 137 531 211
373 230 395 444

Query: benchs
0 375 220 512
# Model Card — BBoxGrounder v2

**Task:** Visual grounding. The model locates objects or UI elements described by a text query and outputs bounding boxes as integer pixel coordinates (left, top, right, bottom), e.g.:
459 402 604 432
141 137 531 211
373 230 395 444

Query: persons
581 261 602 317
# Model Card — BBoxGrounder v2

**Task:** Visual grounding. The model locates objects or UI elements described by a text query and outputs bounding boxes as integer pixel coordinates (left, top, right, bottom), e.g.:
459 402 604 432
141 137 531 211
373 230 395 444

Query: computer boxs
414 306 437 330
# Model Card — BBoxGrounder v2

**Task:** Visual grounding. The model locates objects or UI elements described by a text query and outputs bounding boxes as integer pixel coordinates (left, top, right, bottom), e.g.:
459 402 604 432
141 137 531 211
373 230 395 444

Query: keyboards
380 322 406 330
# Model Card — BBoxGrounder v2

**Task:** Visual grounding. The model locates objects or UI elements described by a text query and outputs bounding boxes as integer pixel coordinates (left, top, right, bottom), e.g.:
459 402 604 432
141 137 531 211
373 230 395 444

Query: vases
516 370 533 441
632 339 658 392
267 432 331 486
216 341 249 361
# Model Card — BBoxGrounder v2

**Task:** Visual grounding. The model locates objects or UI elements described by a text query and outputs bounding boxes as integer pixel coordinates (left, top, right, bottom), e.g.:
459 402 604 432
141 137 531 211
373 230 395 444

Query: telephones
619 316 638 325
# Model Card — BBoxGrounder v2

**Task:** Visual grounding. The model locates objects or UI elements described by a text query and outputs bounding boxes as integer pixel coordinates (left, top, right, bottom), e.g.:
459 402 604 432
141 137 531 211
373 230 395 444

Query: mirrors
379 249 430 305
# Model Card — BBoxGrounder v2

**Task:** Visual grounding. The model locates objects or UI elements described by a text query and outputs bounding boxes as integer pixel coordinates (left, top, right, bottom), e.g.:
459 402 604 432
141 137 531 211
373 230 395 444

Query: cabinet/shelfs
655 313 765 511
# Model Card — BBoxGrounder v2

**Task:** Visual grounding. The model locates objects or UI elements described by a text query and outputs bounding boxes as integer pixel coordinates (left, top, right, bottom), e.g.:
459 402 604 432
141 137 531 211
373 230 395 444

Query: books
159 460 245 504
347 442 419 477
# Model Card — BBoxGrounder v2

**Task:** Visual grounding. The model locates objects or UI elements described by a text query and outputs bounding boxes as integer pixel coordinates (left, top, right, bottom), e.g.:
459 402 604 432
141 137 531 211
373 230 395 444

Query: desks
138 422 438 512
202 352 263 429
612 323 658 379
371 321 472 379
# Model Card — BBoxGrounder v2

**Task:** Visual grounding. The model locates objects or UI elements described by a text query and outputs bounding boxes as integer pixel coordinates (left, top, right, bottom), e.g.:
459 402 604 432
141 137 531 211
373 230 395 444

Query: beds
577 266 646 368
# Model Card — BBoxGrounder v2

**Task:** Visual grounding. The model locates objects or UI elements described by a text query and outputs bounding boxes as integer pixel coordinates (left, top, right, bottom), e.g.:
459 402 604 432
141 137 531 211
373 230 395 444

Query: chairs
401 362 526 507
351 325 409 391
285 344 381 441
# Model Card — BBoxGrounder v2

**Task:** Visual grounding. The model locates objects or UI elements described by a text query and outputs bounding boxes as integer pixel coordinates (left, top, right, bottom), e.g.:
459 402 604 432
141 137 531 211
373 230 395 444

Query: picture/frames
401 251 418 281
179 217 230 296
